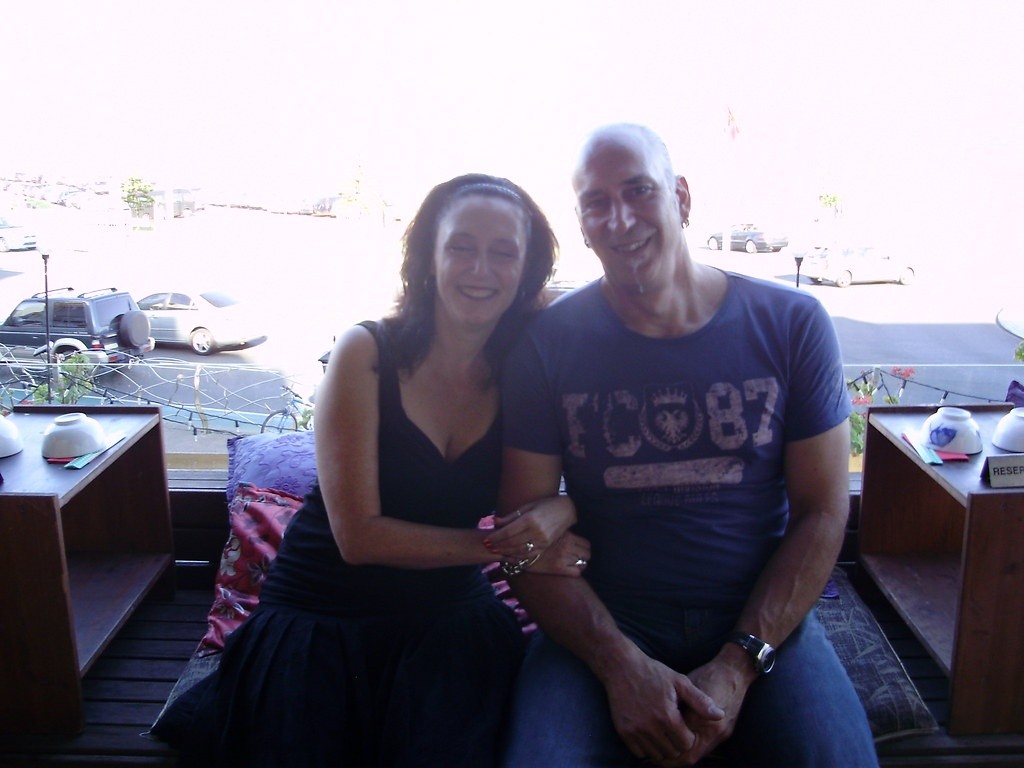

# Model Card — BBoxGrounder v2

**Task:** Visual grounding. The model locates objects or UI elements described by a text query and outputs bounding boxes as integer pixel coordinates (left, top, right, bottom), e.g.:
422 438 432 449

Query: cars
135 289 266 356
707 221 790 256
800 241 919 289
0 216 38 252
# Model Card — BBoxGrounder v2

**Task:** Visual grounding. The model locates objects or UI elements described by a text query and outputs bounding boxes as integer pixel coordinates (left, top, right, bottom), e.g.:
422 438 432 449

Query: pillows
221 435 322 504
194 481 542 660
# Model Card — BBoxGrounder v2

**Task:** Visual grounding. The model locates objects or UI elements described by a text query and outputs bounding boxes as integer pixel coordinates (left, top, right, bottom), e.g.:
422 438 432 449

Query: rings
525 542 534 552
516 509 521 517
575 559 587 566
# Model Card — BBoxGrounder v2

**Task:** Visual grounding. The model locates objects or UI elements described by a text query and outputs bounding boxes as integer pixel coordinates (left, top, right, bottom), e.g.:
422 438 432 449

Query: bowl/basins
42 413 107 458
0 414 23 457
920 406 984 454
991 407 1024 453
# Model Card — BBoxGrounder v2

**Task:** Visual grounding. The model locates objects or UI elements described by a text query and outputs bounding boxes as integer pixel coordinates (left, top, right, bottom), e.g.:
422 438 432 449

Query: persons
505 122 878 768
149 173 592 768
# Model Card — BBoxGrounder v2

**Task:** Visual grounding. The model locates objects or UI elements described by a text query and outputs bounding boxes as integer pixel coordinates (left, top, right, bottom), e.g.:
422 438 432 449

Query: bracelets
502 553 540 577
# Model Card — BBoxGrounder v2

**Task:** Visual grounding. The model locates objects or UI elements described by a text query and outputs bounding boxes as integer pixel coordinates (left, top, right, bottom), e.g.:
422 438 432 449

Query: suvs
0 287 157 368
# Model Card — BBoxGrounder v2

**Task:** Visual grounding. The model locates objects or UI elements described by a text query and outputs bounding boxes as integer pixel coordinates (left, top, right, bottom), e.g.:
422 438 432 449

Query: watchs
720 631 777 675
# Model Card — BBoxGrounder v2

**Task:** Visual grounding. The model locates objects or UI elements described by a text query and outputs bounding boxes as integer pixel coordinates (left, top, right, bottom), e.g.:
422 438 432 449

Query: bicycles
260 384 313 435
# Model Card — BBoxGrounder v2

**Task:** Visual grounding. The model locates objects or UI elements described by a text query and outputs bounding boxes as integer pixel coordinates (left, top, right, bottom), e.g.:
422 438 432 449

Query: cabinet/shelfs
0 404 176 735
851 399 1023 735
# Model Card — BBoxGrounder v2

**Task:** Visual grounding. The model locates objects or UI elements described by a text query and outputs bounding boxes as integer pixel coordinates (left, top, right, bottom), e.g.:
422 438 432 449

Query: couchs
142 430 941 746
